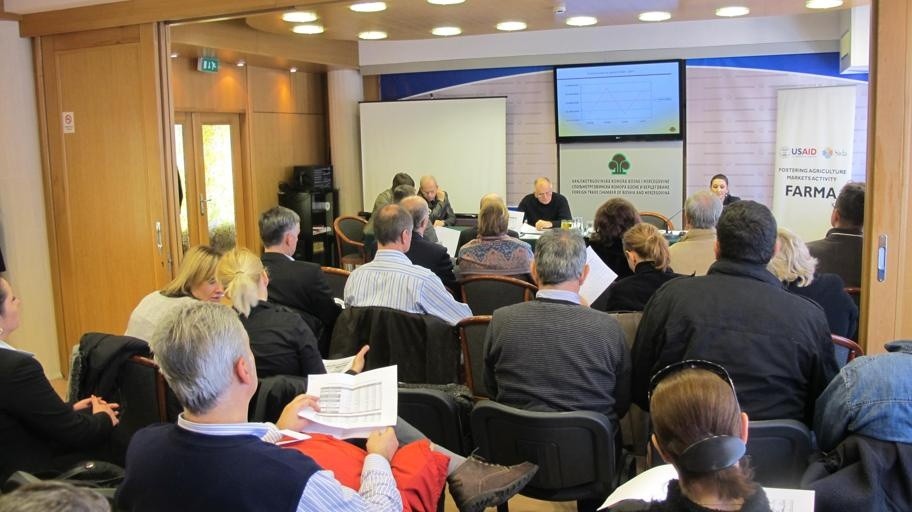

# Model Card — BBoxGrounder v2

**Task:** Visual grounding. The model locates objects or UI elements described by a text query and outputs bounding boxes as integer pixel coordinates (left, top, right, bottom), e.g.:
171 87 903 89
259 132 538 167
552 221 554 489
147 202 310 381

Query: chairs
637 208 674 230
649 417 814 490
102 358 168 434
474 399 617 512
460 272 538 315
844 284 863 306
315 263 350 300
452 312 503 394
391 386 468 448
332 215 368 268
819 333 867 370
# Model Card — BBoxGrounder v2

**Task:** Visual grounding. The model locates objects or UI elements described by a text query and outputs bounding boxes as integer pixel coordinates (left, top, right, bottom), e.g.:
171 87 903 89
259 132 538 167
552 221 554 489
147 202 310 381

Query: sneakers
449 447 539 512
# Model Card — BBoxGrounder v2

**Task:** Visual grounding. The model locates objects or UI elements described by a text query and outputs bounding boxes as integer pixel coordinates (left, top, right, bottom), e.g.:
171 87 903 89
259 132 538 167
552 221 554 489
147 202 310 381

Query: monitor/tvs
553 59 687 142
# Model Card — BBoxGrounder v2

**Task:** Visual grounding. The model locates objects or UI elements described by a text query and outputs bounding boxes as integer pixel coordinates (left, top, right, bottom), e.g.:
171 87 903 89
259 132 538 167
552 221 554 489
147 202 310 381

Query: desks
435 226 687 251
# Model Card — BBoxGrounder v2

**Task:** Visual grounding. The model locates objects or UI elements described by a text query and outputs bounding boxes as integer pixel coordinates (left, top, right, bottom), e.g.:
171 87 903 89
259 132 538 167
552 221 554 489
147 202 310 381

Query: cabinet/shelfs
279 188 338 265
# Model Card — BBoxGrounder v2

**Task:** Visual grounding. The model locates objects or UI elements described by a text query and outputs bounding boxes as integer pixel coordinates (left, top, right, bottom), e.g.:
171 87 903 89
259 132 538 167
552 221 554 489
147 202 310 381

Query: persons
123 245 224 387
595 368 772 512
518 177 572 230
214 246 369 379
0 278 120 477
709 174 740 205
416 174 456 228
589 223 693 313
393 185 439 242
481 228 633 471
633 200 839 429
667 191 723 276
453 193 519 259
399 196 457 290
458 201 534 272
804 181 864 290
585 197 643 311
258 207 341 318
344 204 473 327
362 173 415 259
113 301 538 512
767 228 859 339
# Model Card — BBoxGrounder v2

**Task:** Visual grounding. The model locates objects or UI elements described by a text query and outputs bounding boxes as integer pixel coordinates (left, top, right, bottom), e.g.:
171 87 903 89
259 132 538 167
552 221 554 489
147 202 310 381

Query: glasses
420 209 432 223
647 358 742 428
